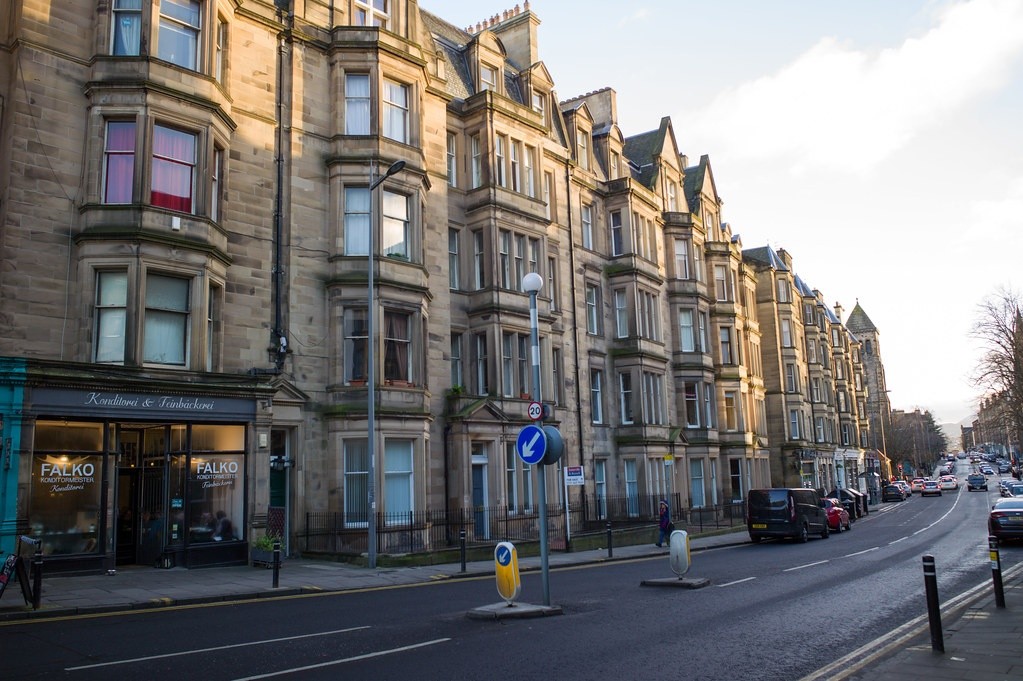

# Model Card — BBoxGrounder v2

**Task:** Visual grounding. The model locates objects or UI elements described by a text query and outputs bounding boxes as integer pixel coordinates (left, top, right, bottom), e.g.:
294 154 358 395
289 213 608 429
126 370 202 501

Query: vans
746 488 830 544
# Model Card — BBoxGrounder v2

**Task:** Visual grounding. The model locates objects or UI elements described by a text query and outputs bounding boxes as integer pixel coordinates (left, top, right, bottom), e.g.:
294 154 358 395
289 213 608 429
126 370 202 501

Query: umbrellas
857 470 880 485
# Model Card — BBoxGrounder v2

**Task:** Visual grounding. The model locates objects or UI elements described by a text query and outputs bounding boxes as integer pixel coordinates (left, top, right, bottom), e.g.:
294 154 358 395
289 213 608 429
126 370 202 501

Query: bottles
89 524 95 532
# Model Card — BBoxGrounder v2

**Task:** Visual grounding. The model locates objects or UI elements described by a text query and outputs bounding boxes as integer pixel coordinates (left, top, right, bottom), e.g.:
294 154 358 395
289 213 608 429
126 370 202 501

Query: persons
212 510 233 540
655 499 670 548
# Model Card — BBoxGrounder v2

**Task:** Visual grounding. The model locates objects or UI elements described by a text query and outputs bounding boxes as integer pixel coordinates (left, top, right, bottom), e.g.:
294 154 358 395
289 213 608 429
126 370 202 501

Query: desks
191 528 213 543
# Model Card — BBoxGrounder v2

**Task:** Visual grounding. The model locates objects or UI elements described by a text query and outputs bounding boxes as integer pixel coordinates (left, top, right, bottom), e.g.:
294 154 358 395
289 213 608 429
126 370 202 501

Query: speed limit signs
528 401 543 420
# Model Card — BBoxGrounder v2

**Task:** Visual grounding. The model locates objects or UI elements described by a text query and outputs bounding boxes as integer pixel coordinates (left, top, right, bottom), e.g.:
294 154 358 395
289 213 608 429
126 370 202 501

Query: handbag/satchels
667 521 674 531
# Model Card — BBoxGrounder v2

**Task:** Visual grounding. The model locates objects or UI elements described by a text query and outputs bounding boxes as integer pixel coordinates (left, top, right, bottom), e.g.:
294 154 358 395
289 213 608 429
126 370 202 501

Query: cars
957 451 1012 474
819 497 852 533
883 480 911 502
965 472 989 491
911 453 959 497
987 476 1023 544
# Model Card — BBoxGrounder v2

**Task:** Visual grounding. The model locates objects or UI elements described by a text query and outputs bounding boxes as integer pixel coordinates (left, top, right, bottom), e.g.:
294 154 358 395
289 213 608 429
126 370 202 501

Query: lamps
260 398 273 410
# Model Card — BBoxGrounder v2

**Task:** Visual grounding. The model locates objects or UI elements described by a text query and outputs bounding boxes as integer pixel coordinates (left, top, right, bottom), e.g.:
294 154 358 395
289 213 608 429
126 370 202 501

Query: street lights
521 272 551 605
367 156 407 566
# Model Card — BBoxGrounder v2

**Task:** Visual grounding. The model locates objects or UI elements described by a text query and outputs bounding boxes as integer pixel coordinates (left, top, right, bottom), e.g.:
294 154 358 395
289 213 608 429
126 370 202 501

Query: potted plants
251 530 287 569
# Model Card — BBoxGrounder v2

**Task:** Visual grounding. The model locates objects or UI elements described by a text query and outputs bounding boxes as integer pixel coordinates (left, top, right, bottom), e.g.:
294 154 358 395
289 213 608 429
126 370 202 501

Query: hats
660 500 668 507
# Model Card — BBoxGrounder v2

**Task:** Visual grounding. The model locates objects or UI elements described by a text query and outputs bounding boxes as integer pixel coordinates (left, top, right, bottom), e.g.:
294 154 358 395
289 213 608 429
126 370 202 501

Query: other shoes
656 543 661 547
